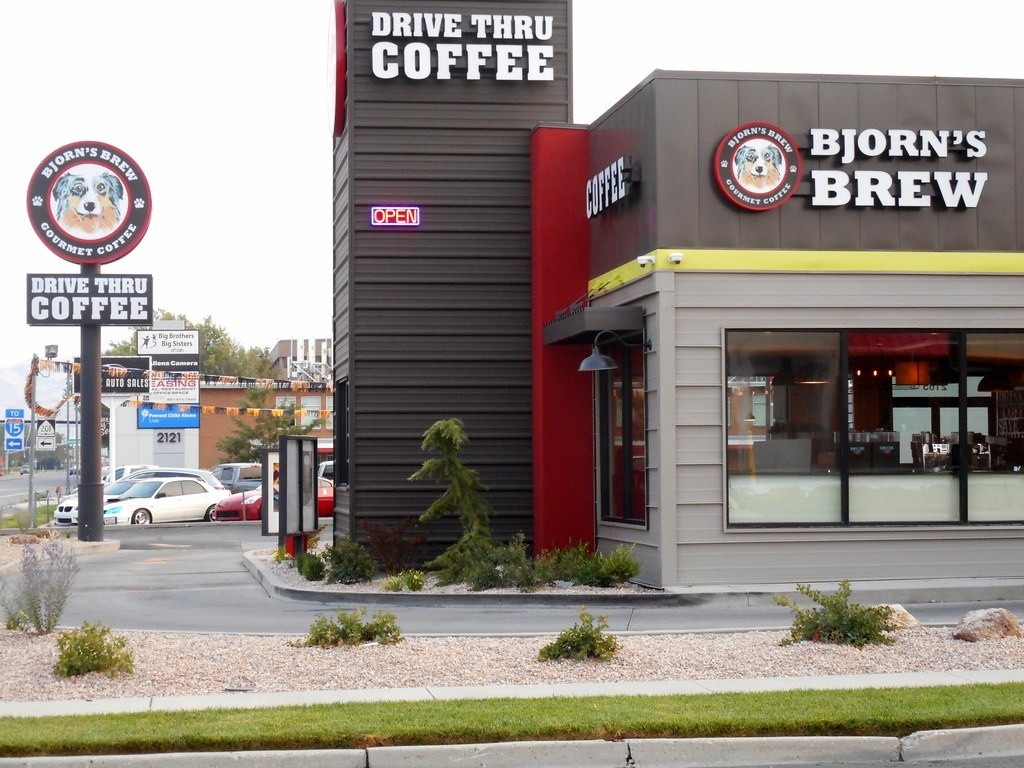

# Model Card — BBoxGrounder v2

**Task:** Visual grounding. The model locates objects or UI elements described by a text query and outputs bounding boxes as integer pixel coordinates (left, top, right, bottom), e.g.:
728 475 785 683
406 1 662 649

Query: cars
70 465 80 474
208 461 263 493
213 475 333 521
316 461 335 482
53 475 232 526
20 465 29 475
103 464 226 492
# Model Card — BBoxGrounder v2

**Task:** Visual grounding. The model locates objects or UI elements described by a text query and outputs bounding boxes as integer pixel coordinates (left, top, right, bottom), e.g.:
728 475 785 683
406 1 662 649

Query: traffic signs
4 408 26 452
35 417 57 453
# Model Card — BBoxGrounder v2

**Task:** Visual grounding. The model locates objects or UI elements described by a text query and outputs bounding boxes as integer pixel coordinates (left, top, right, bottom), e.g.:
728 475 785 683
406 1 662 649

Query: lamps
930 364 959 386
578 331 653 372
896 353 929 386
978 373 1016 393
